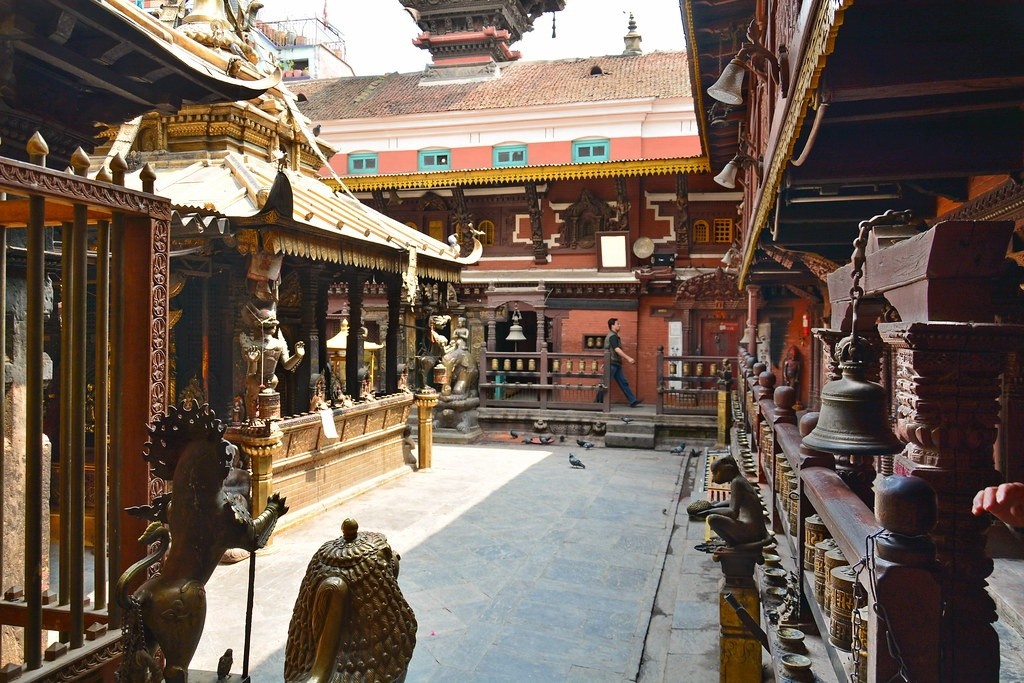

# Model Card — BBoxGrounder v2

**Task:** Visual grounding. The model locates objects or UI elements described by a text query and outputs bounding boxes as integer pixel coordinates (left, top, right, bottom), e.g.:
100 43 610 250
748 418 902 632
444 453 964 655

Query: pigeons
568 453 586 469
690 447 704 458
509 429 596 451
619 416 636 425
669 441 687 455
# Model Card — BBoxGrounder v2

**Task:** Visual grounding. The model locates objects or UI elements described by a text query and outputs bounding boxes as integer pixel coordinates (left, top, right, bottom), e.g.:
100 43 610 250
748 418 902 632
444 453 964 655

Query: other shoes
631 400 644 408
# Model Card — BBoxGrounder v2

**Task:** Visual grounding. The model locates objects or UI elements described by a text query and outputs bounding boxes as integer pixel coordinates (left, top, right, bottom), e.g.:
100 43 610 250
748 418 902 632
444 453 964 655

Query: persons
717 359 732 382
971 480 1024 545
706 453 773 551
593 318 645 408
451 317 469 351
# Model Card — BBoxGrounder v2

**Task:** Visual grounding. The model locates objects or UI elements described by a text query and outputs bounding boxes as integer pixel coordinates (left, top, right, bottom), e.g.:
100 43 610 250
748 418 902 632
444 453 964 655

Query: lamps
705 18 781 104
721 247 742 265
711 147 761 190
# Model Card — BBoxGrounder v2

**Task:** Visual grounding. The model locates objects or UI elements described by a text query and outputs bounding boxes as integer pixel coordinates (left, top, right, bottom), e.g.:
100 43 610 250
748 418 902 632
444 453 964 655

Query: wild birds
216 648 234 681
313 124 321 137
